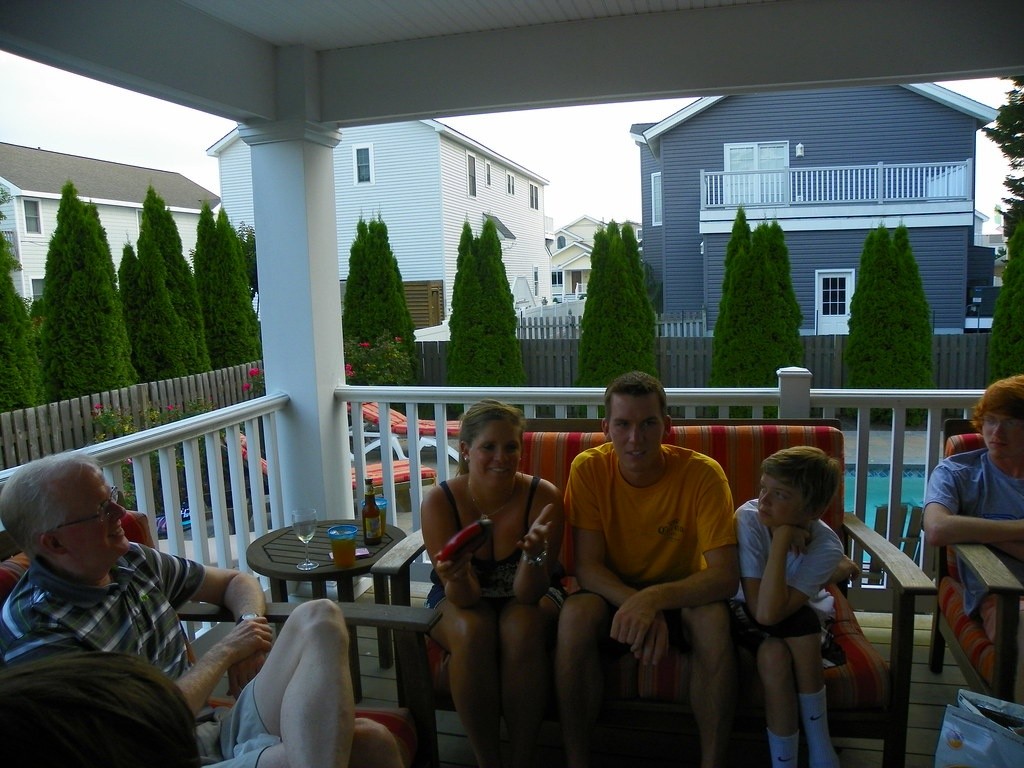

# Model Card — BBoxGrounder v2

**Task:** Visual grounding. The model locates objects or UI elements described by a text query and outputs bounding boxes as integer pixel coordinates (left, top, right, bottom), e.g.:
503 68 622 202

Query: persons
421 399 565 768
0 452 406 768
733 446 860 768
923 374 1024 705
555 371 740 768
0 650 202 768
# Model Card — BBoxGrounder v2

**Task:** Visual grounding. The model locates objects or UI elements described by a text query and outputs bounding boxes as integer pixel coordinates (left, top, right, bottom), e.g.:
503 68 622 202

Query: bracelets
522 541 548 567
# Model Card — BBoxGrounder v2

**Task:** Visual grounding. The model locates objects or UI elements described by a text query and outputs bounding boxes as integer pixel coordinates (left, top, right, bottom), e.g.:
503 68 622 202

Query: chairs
348 402 472 465
927 419 1024 729
219 431 438 512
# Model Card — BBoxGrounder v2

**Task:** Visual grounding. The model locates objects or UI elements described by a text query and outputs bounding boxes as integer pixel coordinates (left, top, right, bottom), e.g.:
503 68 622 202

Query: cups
361 497 388 536
328 525 358 568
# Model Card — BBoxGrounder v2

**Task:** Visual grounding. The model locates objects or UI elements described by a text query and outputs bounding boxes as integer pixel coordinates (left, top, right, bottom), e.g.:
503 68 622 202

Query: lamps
796 142 805 157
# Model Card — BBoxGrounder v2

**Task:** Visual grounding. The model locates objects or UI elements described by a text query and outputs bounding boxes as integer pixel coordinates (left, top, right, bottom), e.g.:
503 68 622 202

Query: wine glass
291 508 319 571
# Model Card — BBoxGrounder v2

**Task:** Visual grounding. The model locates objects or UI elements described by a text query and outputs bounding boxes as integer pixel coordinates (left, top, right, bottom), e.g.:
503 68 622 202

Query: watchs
236 613 259 626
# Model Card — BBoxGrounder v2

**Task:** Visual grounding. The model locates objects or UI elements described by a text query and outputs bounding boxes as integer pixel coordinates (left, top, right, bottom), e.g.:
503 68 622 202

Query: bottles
362 478 382 546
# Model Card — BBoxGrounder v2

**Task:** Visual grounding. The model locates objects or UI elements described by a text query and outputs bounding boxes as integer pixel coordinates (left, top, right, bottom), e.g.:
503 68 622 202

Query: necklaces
468 481 504 520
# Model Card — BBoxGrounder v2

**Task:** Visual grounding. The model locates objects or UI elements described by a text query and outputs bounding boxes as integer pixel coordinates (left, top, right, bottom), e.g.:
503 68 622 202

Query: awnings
483 214 516 241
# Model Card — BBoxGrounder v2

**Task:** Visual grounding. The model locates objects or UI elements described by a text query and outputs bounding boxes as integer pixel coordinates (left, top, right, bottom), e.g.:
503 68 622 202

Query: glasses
980 416 1023 430
54 486 118 530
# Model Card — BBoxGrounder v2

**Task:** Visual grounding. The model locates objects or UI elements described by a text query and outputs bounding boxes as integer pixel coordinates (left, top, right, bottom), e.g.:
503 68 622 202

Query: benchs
0 492 443 767
369 416 934 767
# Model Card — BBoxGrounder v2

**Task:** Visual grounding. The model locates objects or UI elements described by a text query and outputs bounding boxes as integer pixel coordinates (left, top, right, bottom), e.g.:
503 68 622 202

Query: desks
245 516 407 702
